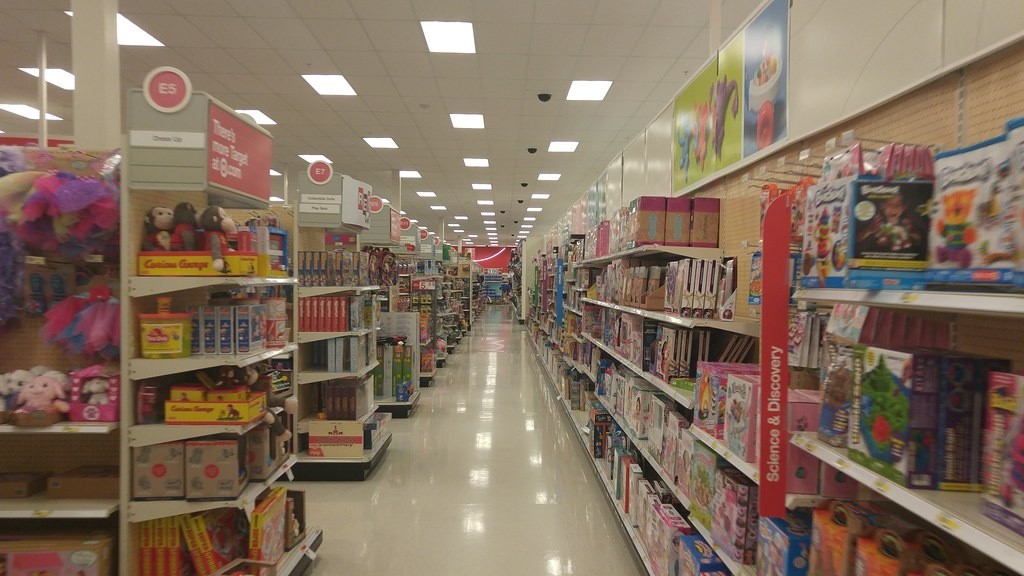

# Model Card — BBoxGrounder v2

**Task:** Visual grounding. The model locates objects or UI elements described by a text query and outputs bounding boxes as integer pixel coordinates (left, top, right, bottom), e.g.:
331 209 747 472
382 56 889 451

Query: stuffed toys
168 202 202 252
141 206 175 252
0 363 110 425
209 363 299 441
200 205 238 271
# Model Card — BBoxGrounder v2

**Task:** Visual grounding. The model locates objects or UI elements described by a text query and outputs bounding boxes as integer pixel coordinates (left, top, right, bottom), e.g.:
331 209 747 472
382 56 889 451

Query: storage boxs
482 275 502 300
298 255 435 458
132 441 186 502
0 473 44 499
201 306 218 354
247 427 282 483
235 304 268 354
186 305 201 355
137 509 249 576
526 196 1024 576
164 385 267 425
135 249 257 276
218 305 235 354
249 487 286 573
185 440 248 501
45 463 121 501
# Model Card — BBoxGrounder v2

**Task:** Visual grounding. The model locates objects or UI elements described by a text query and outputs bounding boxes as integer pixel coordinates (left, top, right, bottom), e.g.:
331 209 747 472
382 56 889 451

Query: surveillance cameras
514 221 518 223
521 183 528 187
512 234 514 236
538 94 551 102
528 148 537 154
501 225 504 227
500 211 505 213
518 200 523 203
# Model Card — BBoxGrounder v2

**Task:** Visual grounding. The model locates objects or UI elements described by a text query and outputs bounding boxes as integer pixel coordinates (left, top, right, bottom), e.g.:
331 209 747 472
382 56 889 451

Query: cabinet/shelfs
791 134 1024 576
0 532 114 576
121 124 322 576
686 423 858 576
581 247 760 576
397 272 437 387
532 264 603 408
0 421 121 518
482 236 543 325
289 213 391 479
438 258 481 366
